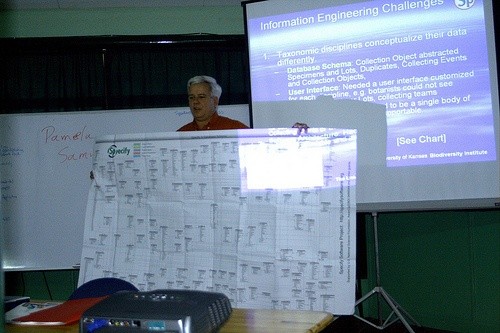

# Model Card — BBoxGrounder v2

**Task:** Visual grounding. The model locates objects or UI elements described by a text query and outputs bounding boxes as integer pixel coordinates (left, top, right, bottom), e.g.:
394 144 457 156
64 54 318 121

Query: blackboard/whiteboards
1 104 250 271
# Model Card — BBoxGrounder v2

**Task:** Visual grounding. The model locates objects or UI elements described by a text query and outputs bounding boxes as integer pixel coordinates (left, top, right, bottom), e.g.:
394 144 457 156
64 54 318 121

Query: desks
6 299 333 333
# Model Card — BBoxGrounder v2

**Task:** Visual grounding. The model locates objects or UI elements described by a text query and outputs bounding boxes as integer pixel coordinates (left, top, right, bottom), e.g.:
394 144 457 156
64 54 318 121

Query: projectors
80 290 232 333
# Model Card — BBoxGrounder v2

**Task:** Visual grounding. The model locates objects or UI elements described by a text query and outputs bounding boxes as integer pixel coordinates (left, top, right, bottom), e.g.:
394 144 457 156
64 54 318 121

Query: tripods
332 213 420 333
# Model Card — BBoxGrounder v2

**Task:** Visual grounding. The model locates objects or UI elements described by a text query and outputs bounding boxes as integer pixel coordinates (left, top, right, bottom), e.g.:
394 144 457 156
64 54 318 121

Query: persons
175 75 308 136
